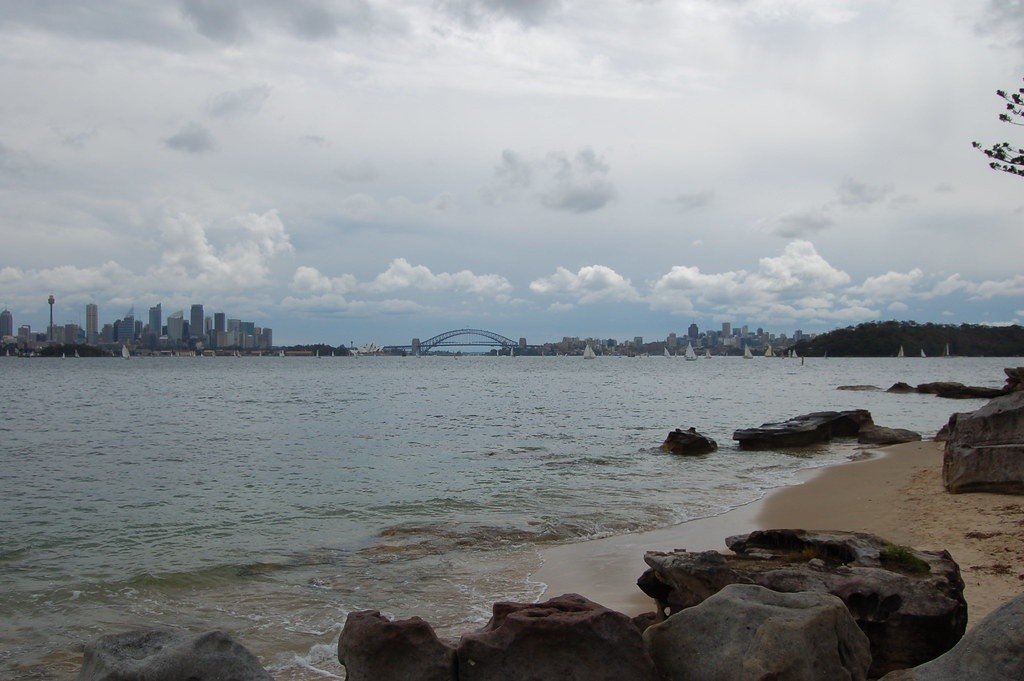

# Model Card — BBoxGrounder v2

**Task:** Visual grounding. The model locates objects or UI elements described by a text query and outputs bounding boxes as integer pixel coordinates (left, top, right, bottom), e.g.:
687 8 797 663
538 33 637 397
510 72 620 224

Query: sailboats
765 345 777 357
685 340 697 362
792 349 798 358
705 349 712 360
744 343 754 359
664 347 671 359
584 344 595 359
